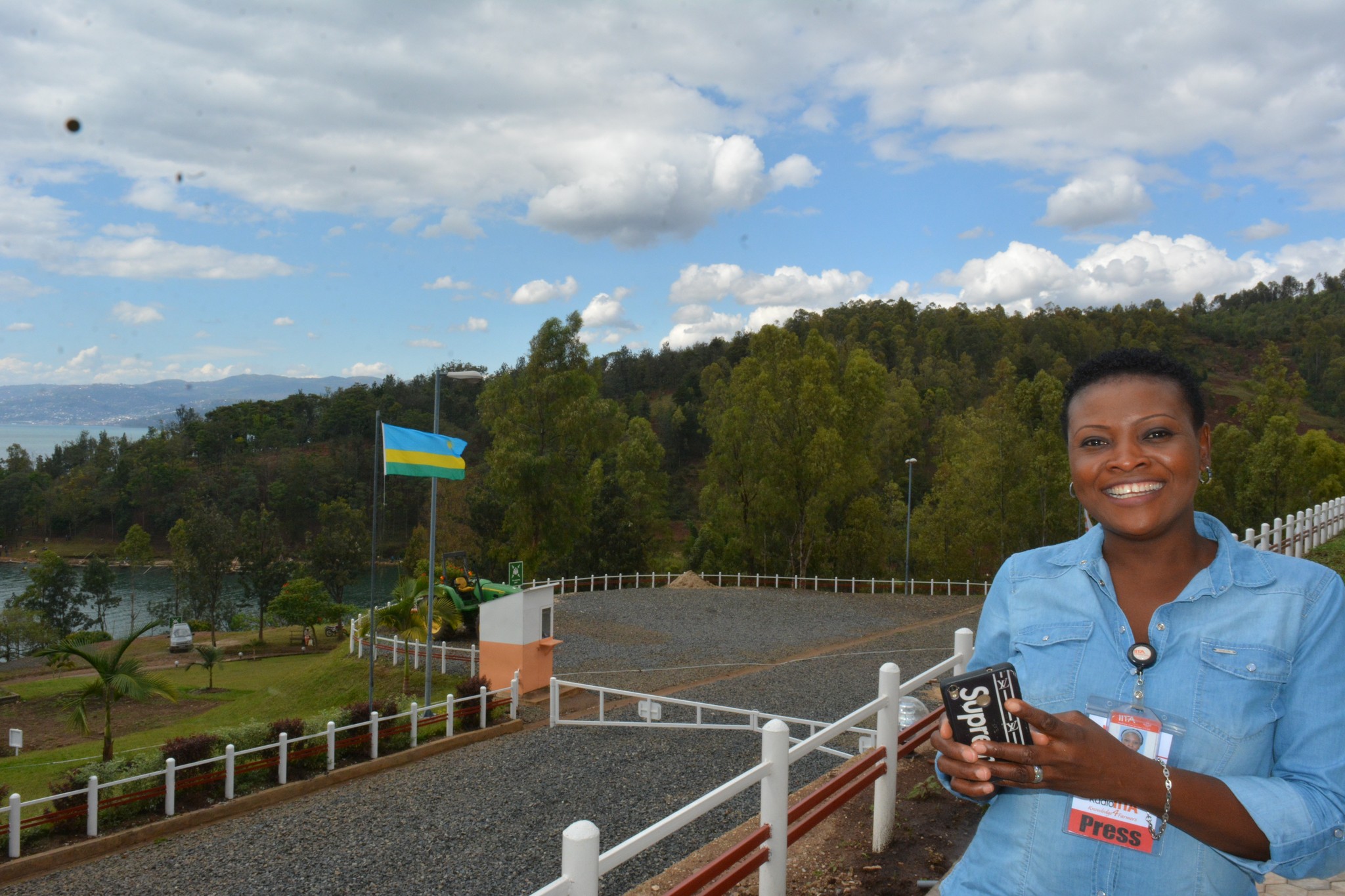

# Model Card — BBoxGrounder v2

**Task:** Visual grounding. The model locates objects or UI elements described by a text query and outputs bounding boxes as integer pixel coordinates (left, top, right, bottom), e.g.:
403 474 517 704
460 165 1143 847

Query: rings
1033 764 1044 783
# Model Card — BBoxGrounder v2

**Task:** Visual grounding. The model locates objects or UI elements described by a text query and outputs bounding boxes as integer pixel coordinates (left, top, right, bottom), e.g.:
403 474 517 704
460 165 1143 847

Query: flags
382 422 468 480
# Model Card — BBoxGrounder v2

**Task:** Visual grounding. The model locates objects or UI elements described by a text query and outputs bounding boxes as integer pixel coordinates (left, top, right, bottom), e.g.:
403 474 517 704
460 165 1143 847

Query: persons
304 627 312 646
926 347 1345 896
1120 728 1144 751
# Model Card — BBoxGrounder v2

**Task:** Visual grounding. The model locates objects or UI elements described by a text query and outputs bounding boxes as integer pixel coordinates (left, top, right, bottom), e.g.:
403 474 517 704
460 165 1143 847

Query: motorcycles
325 623 348 637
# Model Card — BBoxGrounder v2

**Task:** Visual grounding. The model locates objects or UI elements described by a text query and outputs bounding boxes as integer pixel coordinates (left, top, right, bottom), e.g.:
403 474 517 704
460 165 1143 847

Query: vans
165 623 195 653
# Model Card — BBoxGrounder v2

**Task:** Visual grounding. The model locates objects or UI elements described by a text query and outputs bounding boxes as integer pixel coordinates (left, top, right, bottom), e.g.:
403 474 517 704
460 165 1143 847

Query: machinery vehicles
412 551 526 643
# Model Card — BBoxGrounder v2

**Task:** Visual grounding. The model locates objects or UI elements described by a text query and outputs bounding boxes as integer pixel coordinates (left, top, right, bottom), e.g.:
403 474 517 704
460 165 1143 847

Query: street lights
904 457 918 595
423 369 482 719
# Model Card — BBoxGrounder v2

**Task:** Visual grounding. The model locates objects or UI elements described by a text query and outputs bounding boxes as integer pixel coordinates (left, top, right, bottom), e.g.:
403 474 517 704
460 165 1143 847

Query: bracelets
1145 757 1173 840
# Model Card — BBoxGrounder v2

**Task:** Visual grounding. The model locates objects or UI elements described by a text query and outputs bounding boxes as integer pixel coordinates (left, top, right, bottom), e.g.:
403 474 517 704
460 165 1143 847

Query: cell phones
937 662 1034 784
